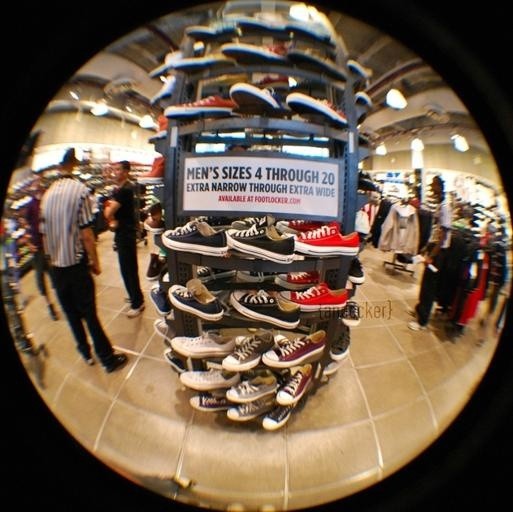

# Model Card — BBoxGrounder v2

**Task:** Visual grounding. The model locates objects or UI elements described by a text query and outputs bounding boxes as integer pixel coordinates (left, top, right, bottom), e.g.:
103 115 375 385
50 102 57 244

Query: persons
38 146 128 373
24 175 60 320
404 174 453 332
104 158 148 319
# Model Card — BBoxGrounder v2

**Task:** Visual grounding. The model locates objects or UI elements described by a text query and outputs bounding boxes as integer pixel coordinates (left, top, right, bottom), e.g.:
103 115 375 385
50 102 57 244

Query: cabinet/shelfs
7 158 150 280
152 22 372 397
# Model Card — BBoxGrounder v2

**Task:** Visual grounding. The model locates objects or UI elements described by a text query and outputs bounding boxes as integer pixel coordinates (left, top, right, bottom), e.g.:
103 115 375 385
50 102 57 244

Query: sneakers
104 353 128 372
124 295 133 302
405 306 418 317
127 302 146 318
408 321 427 330
